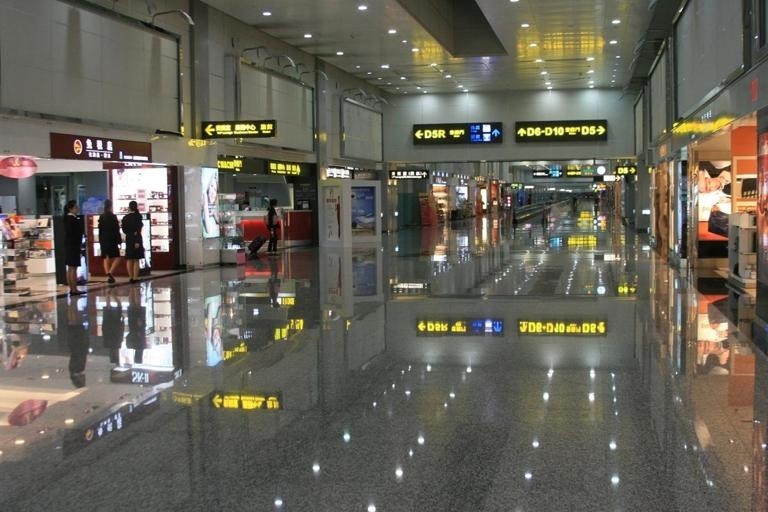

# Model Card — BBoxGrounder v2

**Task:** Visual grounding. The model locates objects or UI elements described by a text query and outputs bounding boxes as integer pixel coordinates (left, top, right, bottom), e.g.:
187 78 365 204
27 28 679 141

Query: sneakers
129 277 141 282
107 273 115 284
70 290 80 295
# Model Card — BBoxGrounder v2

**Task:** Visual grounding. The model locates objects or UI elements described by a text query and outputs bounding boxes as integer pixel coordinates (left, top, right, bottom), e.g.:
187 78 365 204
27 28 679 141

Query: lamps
8 398 50 426
0 157 37 179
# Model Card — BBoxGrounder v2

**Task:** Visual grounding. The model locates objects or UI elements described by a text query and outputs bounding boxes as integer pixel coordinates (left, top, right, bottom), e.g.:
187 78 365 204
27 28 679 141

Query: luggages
248 233 270 254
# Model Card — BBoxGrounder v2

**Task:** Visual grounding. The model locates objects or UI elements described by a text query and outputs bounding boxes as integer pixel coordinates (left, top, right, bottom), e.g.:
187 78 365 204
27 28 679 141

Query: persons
264 199 280 251
697 161 731 196
203 300 226 357
570 193 577 217
64 295 91 389
124 284 148 365
593 195 598 216
264 257 282 308
201 171 221 238
691 338 732 375
98 199 123 284
101 287 125 365
60 199 85 297
121 201 146 282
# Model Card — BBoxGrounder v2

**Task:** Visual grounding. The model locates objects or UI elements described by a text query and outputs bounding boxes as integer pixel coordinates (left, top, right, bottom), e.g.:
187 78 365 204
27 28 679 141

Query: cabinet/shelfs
87 213 153 277
87 271 182 386
2 296 58 336
0 237 30 296
24 227 54 260
727 211 757 289
118 198 178 271
725 283 755 345
